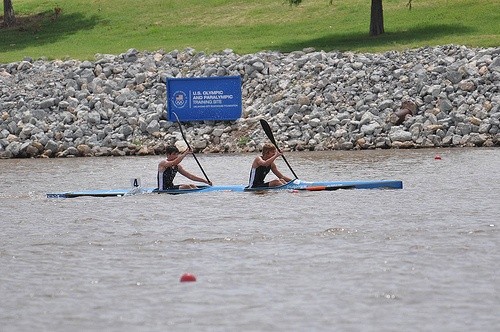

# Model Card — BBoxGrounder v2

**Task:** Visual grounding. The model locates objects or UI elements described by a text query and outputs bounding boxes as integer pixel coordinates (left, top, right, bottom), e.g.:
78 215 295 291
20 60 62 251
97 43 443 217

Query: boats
47 180 403 199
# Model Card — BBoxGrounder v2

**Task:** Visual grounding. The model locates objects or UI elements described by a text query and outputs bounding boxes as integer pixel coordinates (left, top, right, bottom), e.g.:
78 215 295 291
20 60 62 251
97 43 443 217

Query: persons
155 144 212 191
246 143 292 190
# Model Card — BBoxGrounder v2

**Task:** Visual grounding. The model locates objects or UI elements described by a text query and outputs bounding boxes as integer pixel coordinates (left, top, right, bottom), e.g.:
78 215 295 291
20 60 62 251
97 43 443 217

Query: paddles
259 118 299 180
171 111 212 185
